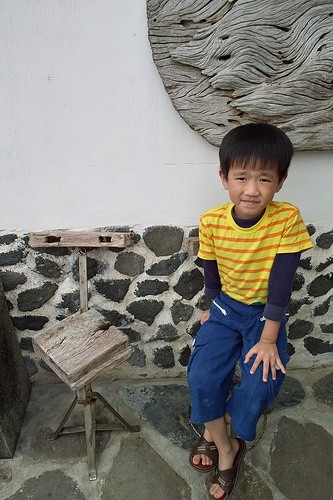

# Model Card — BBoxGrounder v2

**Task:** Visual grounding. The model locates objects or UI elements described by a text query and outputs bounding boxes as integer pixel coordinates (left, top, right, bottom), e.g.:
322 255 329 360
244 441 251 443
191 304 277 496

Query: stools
28 230 135 480
226 363 276 452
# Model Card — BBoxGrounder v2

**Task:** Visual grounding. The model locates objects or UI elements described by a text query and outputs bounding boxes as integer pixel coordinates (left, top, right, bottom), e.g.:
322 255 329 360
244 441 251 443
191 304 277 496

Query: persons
184 121 316 500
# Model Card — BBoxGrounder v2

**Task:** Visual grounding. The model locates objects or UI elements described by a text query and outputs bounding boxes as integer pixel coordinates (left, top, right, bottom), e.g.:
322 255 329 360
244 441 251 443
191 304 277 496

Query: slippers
189 436 218 473
210 437 247 500
